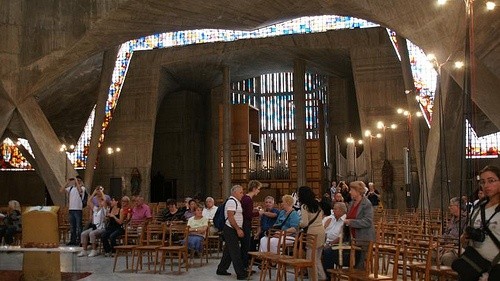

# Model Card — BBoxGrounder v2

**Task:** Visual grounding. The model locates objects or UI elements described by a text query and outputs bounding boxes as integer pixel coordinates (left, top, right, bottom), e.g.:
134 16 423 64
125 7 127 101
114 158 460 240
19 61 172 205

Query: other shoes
104 251 112 257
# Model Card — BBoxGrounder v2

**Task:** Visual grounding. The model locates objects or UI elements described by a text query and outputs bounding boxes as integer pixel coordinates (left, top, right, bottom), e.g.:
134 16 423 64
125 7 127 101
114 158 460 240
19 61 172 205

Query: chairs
0 198 460 281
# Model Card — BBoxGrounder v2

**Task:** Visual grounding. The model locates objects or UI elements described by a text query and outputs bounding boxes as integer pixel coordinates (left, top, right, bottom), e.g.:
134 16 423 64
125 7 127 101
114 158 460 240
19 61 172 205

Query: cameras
466 226 485 242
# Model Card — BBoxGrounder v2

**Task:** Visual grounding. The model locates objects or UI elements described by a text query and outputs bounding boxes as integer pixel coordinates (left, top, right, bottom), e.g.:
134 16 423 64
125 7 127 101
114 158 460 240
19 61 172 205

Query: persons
425 188 484 266
240 181 381 276
216 185 253 280
459 166 500 281
0 200 22 245
60 172 219 257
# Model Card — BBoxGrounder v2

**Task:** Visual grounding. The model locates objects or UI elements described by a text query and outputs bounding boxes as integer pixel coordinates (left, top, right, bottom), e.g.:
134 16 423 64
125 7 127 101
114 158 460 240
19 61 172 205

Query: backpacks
213 197 238 232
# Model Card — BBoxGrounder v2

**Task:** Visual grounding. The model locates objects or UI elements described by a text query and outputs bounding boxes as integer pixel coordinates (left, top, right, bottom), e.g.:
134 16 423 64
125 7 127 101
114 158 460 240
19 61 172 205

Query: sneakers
77 249 88 257
88 248 100 257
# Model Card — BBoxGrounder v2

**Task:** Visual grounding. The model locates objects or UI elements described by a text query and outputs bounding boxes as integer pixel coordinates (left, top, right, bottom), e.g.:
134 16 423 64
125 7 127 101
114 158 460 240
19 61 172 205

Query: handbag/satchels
265 225 282 238
297 229 307 250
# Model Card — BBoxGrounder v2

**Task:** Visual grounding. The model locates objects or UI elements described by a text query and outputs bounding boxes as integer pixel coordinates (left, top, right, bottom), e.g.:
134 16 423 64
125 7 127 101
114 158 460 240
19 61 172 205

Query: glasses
478 178 500 186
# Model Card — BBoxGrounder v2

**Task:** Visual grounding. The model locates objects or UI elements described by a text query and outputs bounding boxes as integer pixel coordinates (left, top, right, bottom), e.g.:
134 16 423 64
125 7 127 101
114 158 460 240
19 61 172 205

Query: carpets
0 269 93 281
